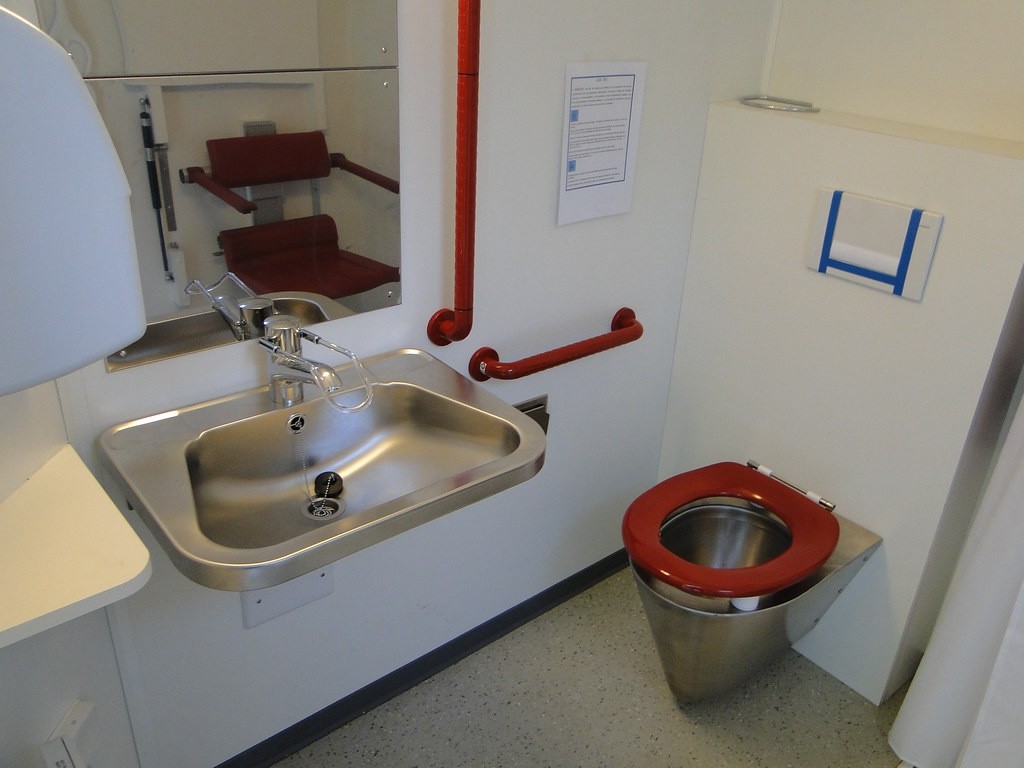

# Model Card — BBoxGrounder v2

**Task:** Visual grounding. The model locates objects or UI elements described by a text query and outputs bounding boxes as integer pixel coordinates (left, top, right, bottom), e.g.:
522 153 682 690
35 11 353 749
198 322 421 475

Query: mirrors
35 0 402 376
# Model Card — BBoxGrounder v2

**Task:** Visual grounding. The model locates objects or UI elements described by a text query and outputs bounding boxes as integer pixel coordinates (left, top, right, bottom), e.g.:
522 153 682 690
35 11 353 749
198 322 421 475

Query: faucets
259 315 372 413
184 271 276 341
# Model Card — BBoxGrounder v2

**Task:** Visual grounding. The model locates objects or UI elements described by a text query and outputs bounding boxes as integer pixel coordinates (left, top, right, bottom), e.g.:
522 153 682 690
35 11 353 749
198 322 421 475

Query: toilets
619 460 884 704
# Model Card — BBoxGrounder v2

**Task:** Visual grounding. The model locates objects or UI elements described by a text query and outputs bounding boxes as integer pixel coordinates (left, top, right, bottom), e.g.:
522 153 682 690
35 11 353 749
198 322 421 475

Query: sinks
113 291 354 360
96 346 546 593
